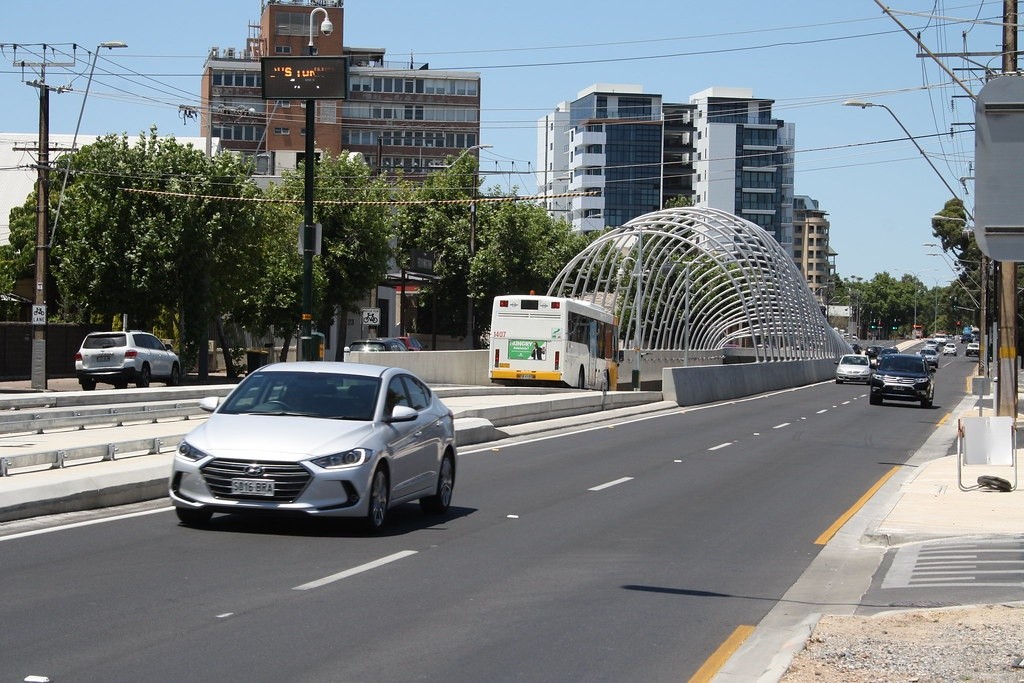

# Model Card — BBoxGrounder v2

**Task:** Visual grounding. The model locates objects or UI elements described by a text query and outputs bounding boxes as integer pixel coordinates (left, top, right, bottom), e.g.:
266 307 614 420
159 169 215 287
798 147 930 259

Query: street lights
432 144 494 349
892 268 939 339
33 40 130 386
840 98 987 377
297 6 334 363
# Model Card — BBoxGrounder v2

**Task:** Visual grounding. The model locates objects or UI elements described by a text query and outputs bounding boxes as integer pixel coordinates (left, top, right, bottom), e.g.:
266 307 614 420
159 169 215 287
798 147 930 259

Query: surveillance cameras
321 21 334 36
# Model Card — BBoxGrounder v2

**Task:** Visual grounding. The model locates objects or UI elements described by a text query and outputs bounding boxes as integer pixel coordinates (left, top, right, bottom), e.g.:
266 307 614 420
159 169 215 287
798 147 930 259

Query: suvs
74 331 183 388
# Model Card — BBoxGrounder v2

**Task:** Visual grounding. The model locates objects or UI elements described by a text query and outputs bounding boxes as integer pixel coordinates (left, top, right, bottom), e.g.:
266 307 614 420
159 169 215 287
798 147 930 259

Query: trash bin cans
245 350 270 376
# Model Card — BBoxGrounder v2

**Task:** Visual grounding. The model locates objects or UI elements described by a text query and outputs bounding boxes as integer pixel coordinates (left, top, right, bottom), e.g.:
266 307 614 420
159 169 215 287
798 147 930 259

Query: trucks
964 343 980 358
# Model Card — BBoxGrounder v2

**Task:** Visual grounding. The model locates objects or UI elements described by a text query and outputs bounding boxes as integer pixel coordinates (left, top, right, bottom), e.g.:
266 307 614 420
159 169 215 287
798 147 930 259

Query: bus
490 291 624 392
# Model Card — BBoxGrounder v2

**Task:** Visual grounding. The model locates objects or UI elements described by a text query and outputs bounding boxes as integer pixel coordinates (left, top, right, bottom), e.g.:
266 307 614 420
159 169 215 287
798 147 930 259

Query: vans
925 339 940 351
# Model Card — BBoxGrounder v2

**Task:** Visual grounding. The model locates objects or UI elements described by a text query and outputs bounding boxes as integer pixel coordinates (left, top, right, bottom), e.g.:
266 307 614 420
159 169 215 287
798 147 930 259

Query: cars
876 347 900 363
864 346 883 357
919 348 940 369
850 344 864 357
943 343 958 356
834 354 873 384
960 334 974 344
168 361 457 531
869 354 936 408
930 333 954 345
346 339 409 353
389 336 427 351
